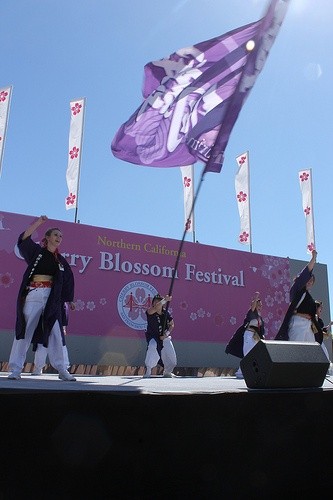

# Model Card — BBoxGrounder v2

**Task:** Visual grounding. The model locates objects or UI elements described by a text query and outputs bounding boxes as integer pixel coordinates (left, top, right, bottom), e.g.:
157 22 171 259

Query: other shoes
143 371 151 378
163 370 176 378
8 370 21 381
59 370 76 382
31 371 42 375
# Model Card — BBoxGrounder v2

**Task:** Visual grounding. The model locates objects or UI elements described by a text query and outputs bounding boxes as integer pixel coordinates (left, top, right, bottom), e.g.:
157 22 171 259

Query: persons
275 250 318 341
29 300 72 379
312 299 332 378
224 291 265 379
5 216 77 382
142 294 176 377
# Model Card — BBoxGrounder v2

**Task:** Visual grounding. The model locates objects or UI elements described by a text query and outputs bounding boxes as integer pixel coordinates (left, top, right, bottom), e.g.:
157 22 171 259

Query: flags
64 99 85 209
234 151 249 247
108 0 292 173
180 165 193 233
297 169 315 254
0 85 13 177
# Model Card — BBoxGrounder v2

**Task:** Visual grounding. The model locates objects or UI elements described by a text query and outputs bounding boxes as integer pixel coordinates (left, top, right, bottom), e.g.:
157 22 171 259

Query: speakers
240 339 330 390
